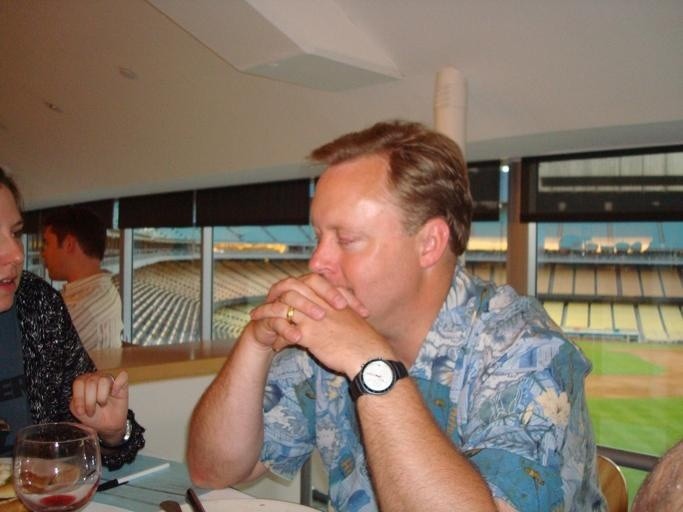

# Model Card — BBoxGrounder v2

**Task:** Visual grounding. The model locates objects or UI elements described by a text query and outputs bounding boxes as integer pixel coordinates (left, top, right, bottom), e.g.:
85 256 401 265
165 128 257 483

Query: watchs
99 420 132 450
348 359 408 402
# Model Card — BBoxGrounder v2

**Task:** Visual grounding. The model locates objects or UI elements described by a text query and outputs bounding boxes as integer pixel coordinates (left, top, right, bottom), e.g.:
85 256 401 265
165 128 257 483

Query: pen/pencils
97 463 169 493
188 489 206 512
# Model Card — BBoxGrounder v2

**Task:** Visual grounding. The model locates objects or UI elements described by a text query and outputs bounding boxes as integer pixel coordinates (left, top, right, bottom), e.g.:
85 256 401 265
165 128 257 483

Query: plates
158 497 322 512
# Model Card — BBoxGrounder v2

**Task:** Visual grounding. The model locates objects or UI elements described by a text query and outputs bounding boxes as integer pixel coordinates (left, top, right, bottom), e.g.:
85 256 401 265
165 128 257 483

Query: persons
41 208 125 378
0 167 145 471
186 121 610 512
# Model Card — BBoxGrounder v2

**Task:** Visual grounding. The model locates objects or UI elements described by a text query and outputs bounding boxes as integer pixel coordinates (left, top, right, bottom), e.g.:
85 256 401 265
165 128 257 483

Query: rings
111 375 115 383
287 306 294 323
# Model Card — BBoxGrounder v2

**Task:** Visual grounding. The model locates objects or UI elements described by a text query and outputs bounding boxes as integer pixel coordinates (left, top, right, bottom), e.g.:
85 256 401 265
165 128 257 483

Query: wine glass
11 420 103 512
